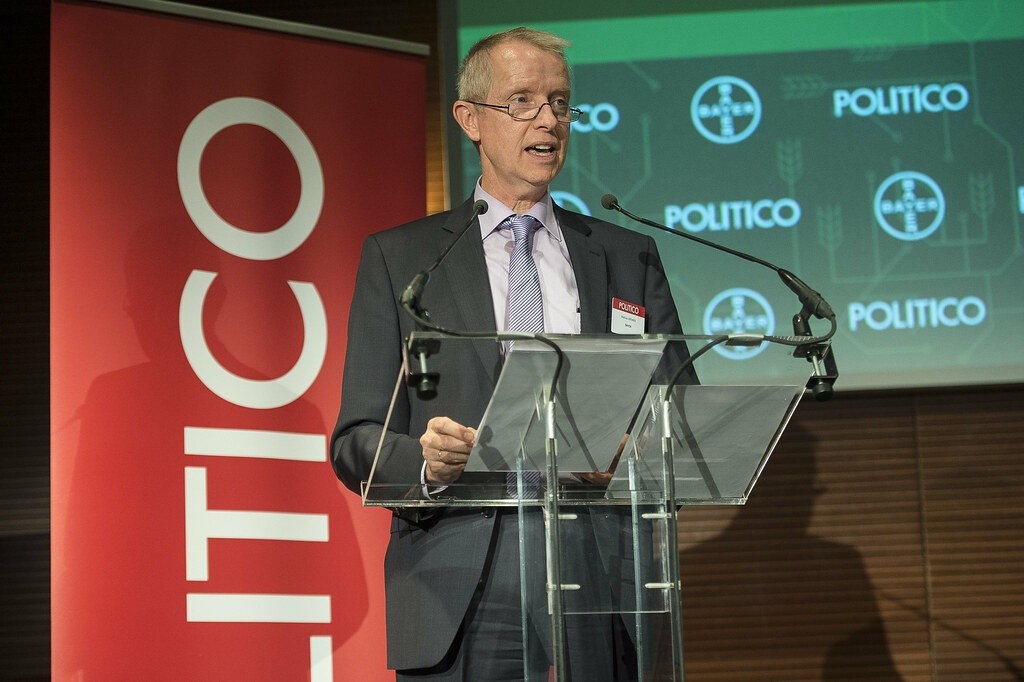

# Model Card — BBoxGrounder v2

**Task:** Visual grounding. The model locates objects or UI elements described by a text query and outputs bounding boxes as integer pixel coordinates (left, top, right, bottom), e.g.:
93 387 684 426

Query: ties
499 215 547 500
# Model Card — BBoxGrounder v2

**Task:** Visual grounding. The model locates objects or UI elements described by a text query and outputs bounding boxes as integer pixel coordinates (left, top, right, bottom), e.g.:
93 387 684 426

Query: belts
502 484 608 500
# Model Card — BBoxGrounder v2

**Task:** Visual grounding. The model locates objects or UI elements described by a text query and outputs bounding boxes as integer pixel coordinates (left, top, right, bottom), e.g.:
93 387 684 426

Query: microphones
401 200 489 309
601 193 837 324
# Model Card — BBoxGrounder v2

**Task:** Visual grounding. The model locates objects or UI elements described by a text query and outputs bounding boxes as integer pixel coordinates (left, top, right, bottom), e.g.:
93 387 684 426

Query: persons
331 26 701 682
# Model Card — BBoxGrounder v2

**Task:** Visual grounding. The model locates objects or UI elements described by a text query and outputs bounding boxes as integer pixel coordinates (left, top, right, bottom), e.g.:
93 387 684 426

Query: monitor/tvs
436 0 1024 401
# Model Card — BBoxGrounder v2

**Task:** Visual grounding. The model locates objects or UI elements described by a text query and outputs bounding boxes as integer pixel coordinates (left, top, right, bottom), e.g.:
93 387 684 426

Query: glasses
464 100 583 123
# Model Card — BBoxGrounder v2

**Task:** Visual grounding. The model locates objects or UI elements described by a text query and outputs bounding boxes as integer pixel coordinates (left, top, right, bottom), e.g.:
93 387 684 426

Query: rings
438 450 442 461
